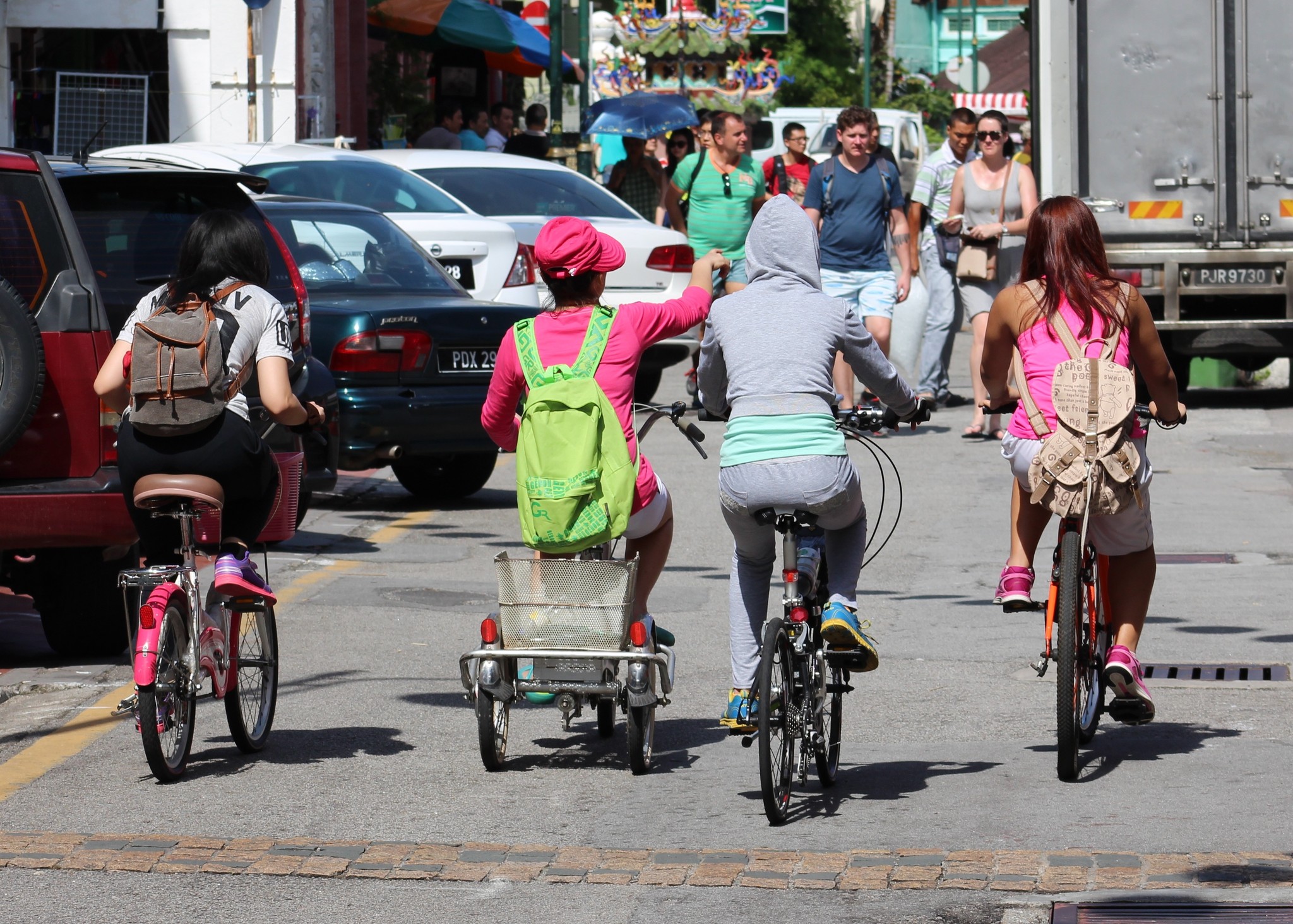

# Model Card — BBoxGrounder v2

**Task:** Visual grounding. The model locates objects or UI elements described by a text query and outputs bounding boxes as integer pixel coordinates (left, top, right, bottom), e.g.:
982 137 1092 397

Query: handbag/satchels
928 216 959 268
679 198 689 230
955 233 1001 281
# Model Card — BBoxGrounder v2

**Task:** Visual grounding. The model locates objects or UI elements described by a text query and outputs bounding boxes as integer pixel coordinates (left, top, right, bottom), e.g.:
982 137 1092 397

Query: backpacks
1012 278 1143 519
129 280 254 437
516 305 641 554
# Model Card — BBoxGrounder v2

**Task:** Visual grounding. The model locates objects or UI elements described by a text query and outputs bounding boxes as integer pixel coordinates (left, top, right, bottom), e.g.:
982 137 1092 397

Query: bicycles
115 397 323 782
697 405 934 825
985 396 1187 781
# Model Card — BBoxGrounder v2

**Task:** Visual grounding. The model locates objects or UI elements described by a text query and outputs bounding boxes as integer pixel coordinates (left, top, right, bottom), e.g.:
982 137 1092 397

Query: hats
534 216 626 279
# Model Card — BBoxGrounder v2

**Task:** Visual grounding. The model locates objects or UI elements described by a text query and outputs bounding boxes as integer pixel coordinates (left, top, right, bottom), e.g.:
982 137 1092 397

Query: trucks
1017 0 1293 408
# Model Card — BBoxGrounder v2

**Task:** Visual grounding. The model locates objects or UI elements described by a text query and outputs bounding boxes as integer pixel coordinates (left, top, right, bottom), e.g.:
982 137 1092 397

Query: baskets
494 550 640 652
192 452 304 543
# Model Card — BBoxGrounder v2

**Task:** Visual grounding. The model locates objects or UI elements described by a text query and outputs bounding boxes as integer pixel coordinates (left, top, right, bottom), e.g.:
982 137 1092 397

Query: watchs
1001 222 1008 234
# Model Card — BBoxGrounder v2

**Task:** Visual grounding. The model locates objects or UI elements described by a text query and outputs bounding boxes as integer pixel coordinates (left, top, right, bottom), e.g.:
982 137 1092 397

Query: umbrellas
579 90 699 140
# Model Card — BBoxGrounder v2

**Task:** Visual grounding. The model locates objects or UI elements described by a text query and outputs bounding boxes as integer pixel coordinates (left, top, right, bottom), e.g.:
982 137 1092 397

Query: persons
832 112 901 177
939 109 1039 440
93 212 326 733
481 215 729 702
980 197 1186 726
762 122 824 238
908 107 975 411
802 106 912 437
698 111 717 149
365 0 665 224
694 193 927 735
655 127 690 231
665 113 765 409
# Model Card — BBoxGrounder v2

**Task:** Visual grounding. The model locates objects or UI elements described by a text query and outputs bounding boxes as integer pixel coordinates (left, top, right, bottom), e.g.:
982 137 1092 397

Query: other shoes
916 392 936 410
857 395 892 436
840 417 859 439
684 368 697 394
693 396 704 408
936 390 969 407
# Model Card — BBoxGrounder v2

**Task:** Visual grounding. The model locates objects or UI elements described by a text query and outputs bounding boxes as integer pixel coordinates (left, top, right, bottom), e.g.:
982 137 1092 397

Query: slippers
986 428 1008 440
961 423 990 439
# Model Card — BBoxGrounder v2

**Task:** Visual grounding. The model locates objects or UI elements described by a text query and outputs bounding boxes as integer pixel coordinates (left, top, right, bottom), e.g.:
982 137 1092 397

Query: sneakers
719 687 759 731
819 601 879 673
993 558 1035 605
1102 645 1155 726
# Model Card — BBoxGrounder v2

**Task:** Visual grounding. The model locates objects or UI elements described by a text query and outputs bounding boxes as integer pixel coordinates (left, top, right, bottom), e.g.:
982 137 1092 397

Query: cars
95 140 542 338
351 147 695 407
749 107 926 214
238 179 529 500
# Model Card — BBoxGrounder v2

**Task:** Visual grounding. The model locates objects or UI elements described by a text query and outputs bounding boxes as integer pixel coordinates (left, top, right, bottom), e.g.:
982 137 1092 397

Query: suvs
50 147 341 544
2 146 119 655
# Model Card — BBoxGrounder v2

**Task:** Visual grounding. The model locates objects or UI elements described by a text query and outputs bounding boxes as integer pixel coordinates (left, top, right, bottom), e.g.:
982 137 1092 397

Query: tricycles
458 395 707 772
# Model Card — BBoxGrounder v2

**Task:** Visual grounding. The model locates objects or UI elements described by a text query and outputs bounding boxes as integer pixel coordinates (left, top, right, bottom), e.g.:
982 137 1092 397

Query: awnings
950 92 1029 125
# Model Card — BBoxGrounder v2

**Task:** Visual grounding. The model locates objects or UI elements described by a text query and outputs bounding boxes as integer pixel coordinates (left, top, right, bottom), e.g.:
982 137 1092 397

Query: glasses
722 173 730 195
697 130 713 139
787 136 809 143
667 140 687 148
975 130 1002 141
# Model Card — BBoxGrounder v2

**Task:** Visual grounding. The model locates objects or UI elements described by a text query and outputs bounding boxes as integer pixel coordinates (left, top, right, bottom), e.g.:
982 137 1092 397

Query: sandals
214 551 277 606
655 625 675 646
134 692 174 734
517 664 557 705
